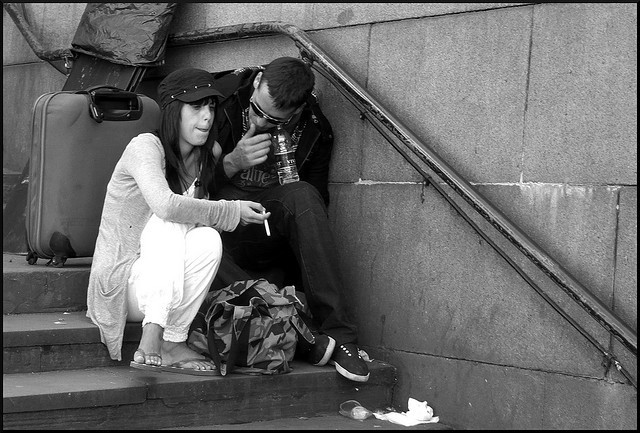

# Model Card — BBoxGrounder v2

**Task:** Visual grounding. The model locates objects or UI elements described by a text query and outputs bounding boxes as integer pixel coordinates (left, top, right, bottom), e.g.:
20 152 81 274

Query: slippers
129 348 163 374
160 353 221 377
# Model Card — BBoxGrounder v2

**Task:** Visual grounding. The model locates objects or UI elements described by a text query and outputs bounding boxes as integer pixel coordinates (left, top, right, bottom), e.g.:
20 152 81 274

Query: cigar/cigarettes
262 210 272 238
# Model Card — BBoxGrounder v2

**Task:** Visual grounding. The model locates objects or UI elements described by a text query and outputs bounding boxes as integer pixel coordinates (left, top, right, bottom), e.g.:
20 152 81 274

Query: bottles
270 123 300 185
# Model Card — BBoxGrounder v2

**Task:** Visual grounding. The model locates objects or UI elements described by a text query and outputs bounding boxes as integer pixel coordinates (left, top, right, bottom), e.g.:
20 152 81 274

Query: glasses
249 91 293 127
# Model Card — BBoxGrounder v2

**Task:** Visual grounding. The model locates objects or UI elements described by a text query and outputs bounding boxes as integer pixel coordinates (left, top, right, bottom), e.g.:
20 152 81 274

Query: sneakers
298 327 336 367
329 342 371 383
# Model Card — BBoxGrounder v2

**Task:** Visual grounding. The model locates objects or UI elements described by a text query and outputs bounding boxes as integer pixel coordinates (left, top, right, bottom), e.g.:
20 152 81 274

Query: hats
157 67 226 108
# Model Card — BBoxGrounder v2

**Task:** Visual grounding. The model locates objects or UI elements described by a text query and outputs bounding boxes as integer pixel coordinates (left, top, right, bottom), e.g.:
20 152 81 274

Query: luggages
22 84 163 268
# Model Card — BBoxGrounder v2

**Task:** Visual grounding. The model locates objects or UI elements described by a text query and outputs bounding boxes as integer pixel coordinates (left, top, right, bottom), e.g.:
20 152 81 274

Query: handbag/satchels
187 277 316 378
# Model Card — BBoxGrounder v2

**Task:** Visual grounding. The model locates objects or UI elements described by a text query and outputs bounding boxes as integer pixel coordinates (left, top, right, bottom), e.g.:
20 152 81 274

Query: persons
84 67 273 376
210 56 370 384
